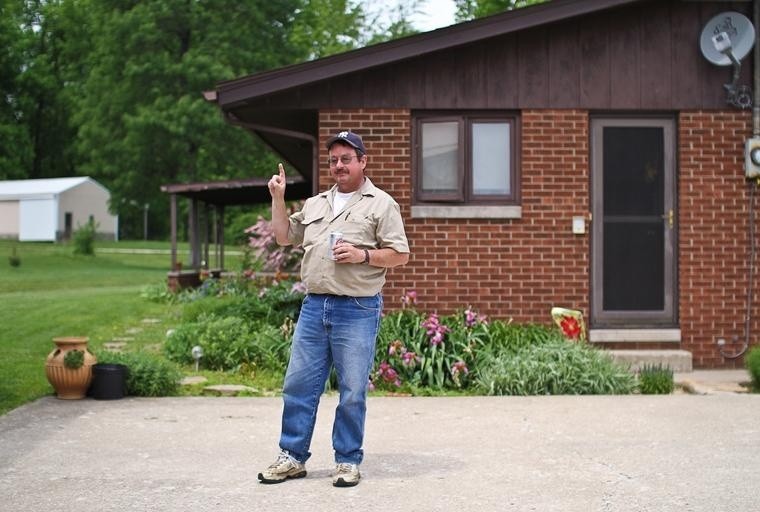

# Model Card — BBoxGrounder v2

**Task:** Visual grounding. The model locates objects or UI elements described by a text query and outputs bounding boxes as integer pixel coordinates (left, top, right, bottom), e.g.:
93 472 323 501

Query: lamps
699 11 757 111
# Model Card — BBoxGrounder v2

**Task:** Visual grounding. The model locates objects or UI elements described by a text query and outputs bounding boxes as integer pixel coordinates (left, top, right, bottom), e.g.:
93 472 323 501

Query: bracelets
360 249 370 265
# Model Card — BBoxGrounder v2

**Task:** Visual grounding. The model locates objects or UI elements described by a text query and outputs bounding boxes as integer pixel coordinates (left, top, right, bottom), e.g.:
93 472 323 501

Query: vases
43 336 97 400
91 363 127 400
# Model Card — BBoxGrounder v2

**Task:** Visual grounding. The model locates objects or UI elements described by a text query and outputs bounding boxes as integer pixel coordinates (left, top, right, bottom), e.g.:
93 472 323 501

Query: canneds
328 233 342 260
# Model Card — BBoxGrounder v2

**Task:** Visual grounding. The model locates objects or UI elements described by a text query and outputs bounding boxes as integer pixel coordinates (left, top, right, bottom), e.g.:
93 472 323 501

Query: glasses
328 153 362 167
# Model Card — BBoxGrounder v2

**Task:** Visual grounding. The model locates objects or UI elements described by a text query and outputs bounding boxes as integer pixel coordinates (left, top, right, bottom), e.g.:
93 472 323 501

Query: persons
258 132 410 486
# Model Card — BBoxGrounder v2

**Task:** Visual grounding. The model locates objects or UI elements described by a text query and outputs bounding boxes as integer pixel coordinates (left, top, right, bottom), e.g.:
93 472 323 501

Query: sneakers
332 463 361 486
258 449 307 483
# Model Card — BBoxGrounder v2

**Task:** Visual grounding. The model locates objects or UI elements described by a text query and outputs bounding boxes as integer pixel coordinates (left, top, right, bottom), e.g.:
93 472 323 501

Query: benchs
167 268 226 294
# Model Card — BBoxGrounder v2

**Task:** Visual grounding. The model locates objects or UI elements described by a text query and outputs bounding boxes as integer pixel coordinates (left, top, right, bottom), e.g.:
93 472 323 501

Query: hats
325 130 366 154
